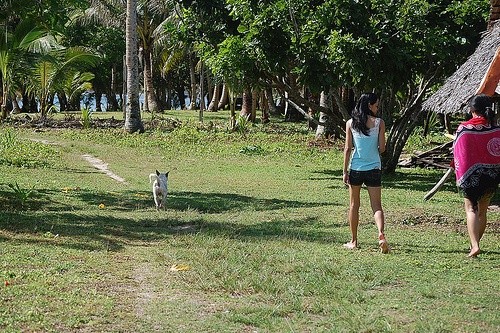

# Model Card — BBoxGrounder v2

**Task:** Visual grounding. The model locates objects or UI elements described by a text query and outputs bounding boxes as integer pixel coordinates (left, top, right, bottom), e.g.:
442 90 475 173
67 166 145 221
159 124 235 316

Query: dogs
149 169 171 208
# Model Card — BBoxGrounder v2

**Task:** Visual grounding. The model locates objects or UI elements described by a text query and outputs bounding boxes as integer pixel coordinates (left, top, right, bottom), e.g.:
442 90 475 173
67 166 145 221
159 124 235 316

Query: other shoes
341 242 358 250
379 239 389 254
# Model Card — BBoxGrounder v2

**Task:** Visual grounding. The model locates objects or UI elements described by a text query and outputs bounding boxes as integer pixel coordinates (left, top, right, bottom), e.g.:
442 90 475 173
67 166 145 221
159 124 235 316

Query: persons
342 92 389 254
450 95 500 258
